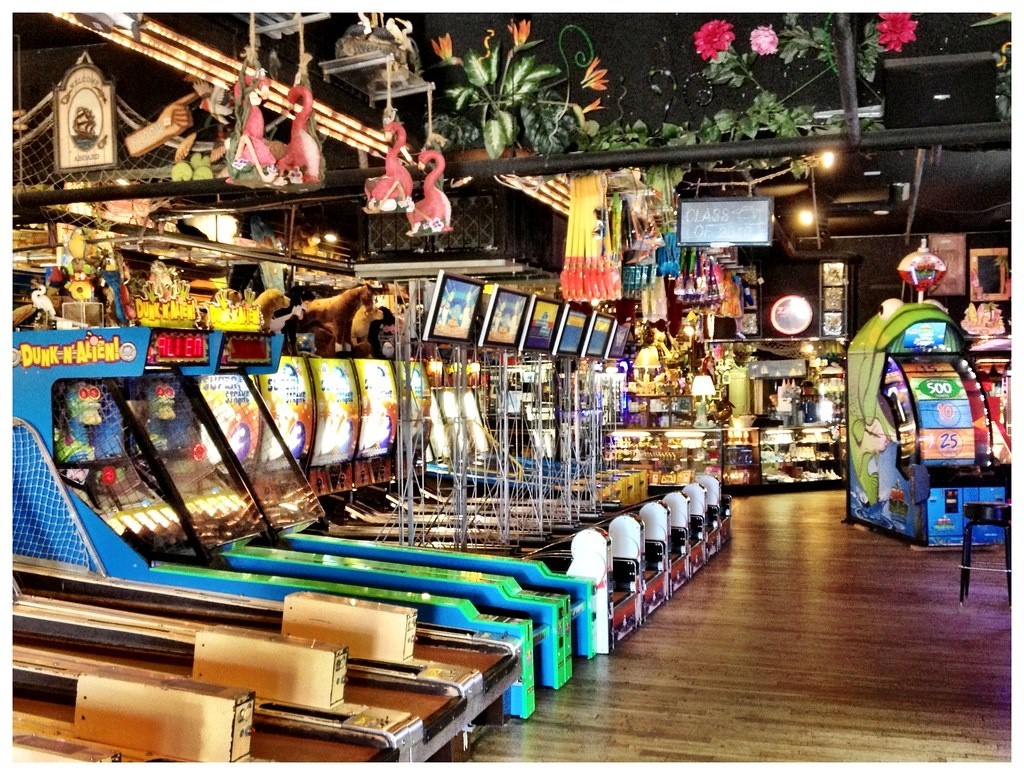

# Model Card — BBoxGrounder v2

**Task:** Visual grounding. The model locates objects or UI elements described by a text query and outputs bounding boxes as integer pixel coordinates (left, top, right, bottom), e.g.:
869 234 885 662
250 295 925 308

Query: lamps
633 347 661 394
691 376 716 428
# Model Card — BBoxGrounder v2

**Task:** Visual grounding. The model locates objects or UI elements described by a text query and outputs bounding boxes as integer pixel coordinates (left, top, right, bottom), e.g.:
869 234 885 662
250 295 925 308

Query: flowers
431 18 610 159
579 15 918 155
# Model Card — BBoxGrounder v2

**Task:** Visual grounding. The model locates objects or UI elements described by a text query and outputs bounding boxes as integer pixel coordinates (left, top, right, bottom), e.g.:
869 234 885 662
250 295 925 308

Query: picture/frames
970 248 1012 302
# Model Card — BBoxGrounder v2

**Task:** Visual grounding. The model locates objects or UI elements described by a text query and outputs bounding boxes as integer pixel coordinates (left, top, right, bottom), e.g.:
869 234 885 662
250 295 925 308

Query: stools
960 502 1011 606
